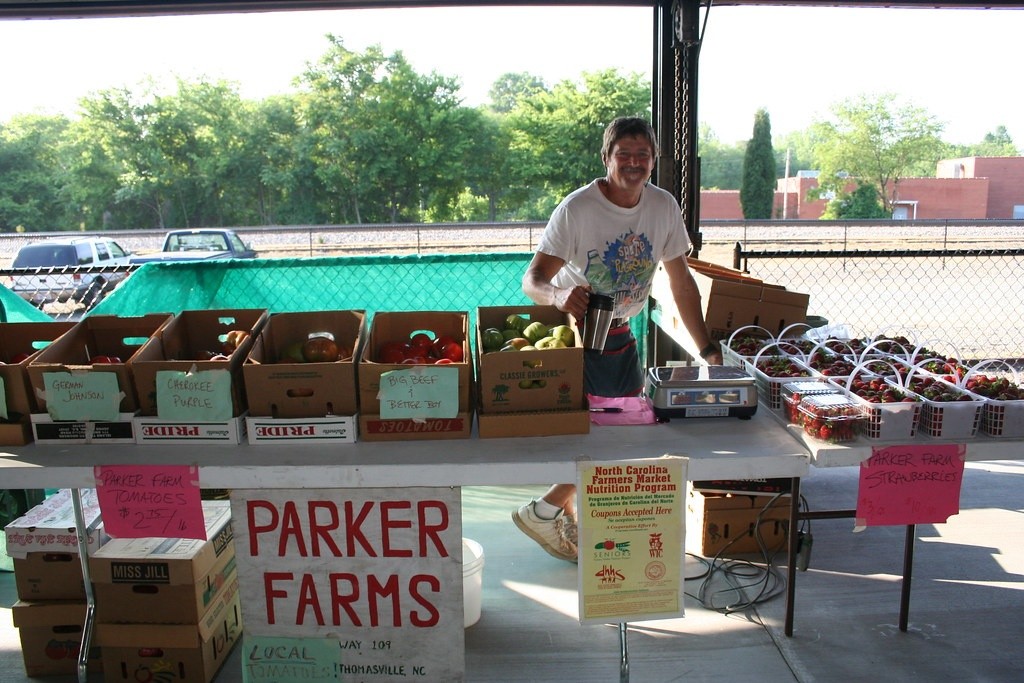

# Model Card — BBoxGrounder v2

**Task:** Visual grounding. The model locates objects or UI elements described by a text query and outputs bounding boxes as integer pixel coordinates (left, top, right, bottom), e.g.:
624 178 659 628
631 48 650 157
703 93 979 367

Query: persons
510 116 723 565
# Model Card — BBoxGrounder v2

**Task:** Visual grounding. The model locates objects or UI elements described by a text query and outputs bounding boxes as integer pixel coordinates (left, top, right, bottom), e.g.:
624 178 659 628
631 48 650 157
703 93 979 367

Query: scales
643 365 759 423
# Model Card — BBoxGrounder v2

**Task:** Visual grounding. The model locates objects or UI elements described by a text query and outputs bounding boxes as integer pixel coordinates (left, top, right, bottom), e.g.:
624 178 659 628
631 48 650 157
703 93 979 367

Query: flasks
576 289 615 356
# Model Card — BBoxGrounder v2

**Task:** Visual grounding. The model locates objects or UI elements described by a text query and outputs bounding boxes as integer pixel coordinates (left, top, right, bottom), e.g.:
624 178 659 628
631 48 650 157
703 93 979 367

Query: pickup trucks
128 228 259 274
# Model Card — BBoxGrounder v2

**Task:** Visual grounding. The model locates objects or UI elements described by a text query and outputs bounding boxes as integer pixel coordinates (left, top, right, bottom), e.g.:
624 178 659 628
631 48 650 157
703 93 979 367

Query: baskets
718 323 1024 444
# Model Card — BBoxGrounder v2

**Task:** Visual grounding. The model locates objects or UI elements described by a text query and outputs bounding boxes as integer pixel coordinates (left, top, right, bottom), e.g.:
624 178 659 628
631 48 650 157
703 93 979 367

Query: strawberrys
727 334 1024 442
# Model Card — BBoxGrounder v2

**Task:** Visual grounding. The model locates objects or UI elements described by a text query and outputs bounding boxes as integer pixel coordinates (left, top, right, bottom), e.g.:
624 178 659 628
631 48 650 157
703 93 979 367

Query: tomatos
9 352 30 364
274 336 352 396
377 334 463 364
87 354 121 365
479 315 575 387
193 329 248 361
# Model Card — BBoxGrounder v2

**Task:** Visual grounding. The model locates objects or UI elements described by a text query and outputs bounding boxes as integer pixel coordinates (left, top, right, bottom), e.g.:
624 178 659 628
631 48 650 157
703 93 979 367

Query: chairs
210 244 223 250
173 246 189 251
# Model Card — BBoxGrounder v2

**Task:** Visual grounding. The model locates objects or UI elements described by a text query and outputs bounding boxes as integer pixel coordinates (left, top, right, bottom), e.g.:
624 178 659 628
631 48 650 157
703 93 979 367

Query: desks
756 396 1024 683
0 399 813 683
0 309 1024 683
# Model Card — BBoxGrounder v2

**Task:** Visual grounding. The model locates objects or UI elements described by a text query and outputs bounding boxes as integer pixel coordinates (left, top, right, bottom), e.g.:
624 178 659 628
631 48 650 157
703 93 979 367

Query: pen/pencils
590 408 623 412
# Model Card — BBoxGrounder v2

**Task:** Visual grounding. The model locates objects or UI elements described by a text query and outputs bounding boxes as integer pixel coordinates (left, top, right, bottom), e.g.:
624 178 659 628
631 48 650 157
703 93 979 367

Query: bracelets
700 344 717 358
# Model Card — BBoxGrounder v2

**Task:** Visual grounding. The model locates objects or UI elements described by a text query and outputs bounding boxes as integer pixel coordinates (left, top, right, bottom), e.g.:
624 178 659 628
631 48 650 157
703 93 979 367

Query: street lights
783 147 793 220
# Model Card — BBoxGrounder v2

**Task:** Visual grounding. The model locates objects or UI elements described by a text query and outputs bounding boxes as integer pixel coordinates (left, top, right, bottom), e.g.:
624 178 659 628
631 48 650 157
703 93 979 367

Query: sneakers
511 500 579 561
560 514 579 565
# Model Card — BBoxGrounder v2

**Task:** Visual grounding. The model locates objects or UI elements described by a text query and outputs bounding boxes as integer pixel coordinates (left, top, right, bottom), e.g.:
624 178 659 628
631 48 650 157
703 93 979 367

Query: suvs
8 237 137 311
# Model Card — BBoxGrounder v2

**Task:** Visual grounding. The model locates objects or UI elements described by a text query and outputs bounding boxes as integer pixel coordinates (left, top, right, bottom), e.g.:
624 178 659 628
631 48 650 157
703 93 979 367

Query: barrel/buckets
463 537 485 629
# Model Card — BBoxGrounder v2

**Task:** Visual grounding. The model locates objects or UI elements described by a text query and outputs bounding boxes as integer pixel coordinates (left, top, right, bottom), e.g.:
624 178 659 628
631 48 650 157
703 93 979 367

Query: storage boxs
26 312 175 413
0 415 34 446
89 571 243 683
0 322 78 424
359 406 473 442
474 305 585 414
5 487 111 559
12 600 103 677
132 307 268 417
359 310 471 416
245 411 358 445
30 408 140 445
93 540 236 625
687 480 792 557
88 500 232 585
133 408 249 445
242 310 367 419
651 257 810 349
476 398 590 439
12 552 95 601
